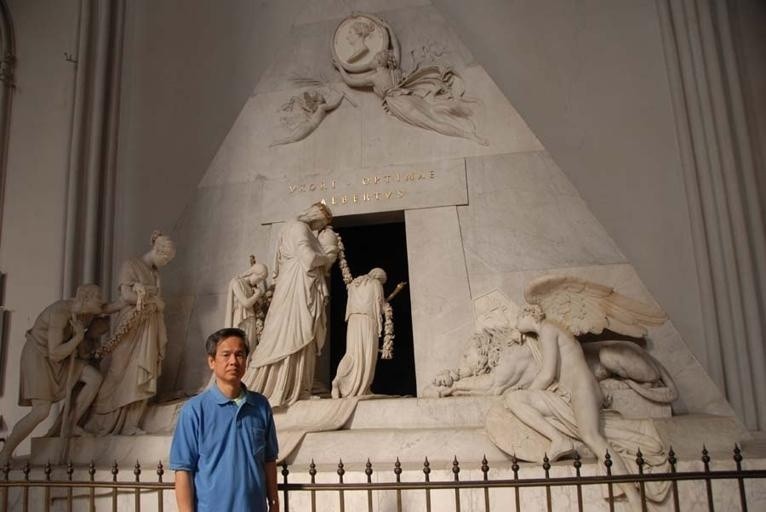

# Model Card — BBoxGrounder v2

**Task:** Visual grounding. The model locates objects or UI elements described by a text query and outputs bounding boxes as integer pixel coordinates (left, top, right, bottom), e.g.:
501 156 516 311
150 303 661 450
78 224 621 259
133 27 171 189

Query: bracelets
269 499 279 506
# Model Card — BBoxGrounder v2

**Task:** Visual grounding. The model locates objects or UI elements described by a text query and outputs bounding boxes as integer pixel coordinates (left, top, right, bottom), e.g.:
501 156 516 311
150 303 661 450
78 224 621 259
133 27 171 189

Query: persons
225 202 387 409
168 328 279 512
506 303 642 512
329 22 488 146
269 91 344 149
